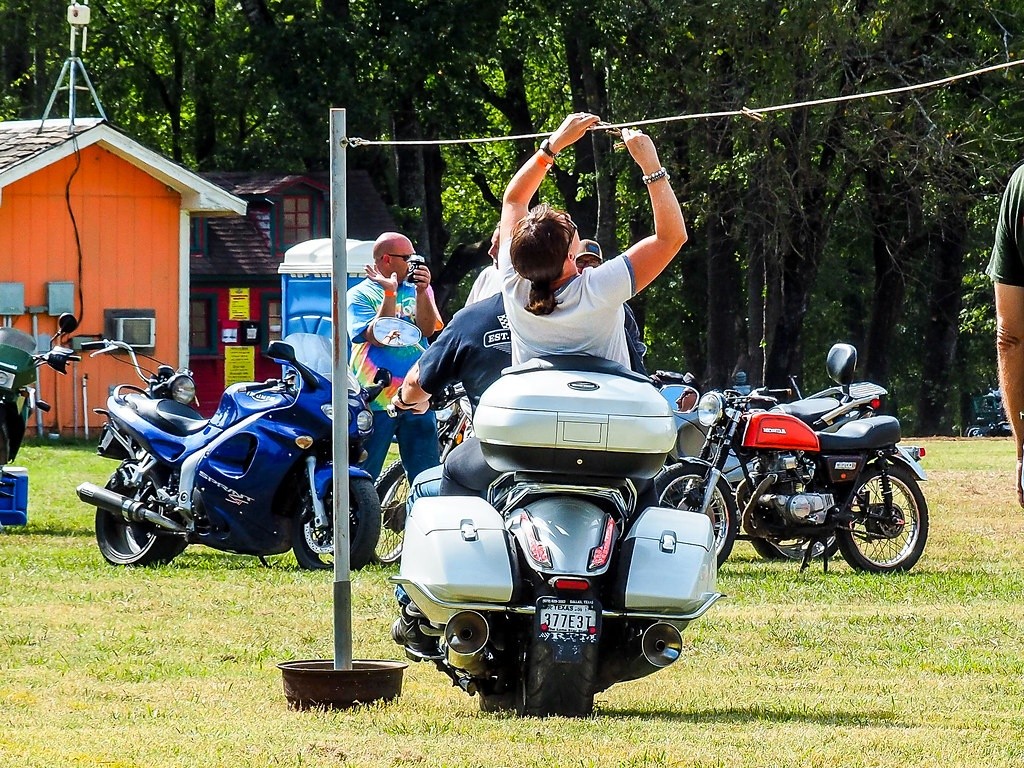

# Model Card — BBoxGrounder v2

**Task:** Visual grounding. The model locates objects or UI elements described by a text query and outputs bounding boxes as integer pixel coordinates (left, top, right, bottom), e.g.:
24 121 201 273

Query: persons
392 291 649 653
676 387 697 412
465 221 503 307
985 164 1024 509
346 232 444 489
575 239 649 377
392 112 687 638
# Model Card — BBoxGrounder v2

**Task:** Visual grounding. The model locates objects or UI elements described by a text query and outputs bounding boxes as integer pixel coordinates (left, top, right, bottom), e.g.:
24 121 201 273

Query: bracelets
534 152 553 170
385 335 391 340
385 291 396 297
642 168 670 183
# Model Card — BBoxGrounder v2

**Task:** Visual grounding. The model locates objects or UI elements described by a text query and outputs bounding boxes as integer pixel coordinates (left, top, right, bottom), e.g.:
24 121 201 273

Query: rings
637 129 642 133
580 112 584 116
580 116 584 119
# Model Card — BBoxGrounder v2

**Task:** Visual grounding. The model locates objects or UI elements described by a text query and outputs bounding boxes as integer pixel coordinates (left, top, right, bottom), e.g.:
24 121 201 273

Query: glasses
679 391 695 401
379 252 416 262
563 217 577 257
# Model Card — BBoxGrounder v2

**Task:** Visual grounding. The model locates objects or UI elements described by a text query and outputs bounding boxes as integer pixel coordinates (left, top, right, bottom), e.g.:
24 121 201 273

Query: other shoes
391 605 437 652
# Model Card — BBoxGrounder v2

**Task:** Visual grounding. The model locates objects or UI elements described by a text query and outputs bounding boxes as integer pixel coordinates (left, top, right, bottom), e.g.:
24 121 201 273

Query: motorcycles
76 333 383 571
1 311 78 467
654 343 929 572
387 351 728 718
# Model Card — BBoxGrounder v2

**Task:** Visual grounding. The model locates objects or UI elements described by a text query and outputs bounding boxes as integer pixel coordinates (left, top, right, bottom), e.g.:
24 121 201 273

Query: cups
407 259 426 283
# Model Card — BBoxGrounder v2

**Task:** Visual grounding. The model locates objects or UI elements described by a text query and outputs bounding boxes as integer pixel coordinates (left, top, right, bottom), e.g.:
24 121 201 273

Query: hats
574 239 603 261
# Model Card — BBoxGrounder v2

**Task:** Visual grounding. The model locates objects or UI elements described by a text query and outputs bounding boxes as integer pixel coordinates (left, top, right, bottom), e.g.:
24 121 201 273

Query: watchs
396 387 417 406
539 139 558 158
